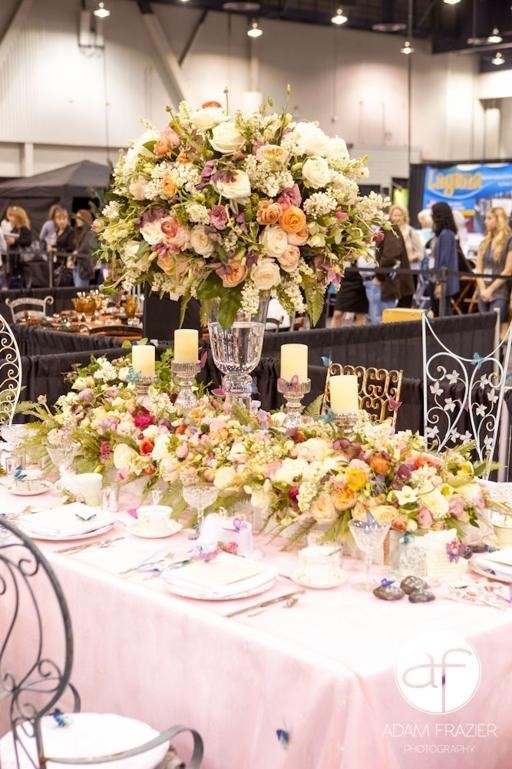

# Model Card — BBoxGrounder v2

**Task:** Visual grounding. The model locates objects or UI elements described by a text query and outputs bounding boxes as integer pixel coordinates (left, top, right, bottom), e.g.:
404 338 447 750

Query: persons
1 201 113 293
332 180 511 326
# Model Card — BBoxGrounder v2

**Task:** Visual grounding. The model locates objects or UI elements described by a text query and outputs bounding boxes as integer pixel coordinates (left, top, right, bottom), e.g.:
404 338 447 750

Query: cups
77 473 103 507
137 505 173 530
300 547 340 580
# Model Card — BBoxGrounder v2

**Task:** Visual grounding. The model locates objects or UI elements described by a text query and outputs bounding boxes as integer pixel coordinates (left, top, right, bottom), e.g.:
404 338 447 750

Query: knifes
54 538 123 553
226 591 301 617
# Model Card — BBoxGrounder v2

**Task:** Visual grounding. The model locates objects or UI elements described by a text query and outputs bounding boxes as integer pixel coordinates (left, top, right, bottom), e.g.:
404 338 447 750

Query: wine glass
46 443 75 490
72 291 139 328
348 517 392 591
183 482 219 543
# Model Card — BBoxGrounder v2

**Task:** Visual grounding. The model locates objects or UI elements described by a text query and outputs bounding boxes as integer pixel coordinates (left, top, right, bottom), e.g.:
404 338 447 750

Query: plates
20 506 114 541
166 562 276 601
466 555 512 585
126 521 182 539
292 570 346 589
8 480 53 496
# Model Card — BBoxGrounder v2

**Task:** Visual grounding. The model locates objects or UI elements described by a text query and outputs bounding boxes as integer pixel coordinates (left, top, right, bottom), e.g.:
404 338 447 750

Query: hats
72 210 93 225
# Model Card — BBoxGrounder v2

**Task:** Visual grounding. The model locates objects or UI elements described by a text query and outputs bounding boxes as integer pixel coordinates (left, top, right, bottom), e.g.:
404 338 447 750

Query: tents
2 158 126 288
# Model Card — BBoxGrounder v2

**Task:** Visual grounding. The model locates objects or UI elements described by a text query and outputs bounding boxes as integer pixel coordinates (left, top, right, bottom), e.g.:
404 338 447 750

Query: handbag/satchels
458 251 472 285
57 267 74 286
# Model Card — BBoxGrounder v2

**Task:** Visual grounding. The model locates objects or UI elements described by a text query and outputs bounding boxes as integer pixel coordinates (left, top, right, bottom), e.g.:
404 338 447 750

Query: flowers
90 81 401 331
